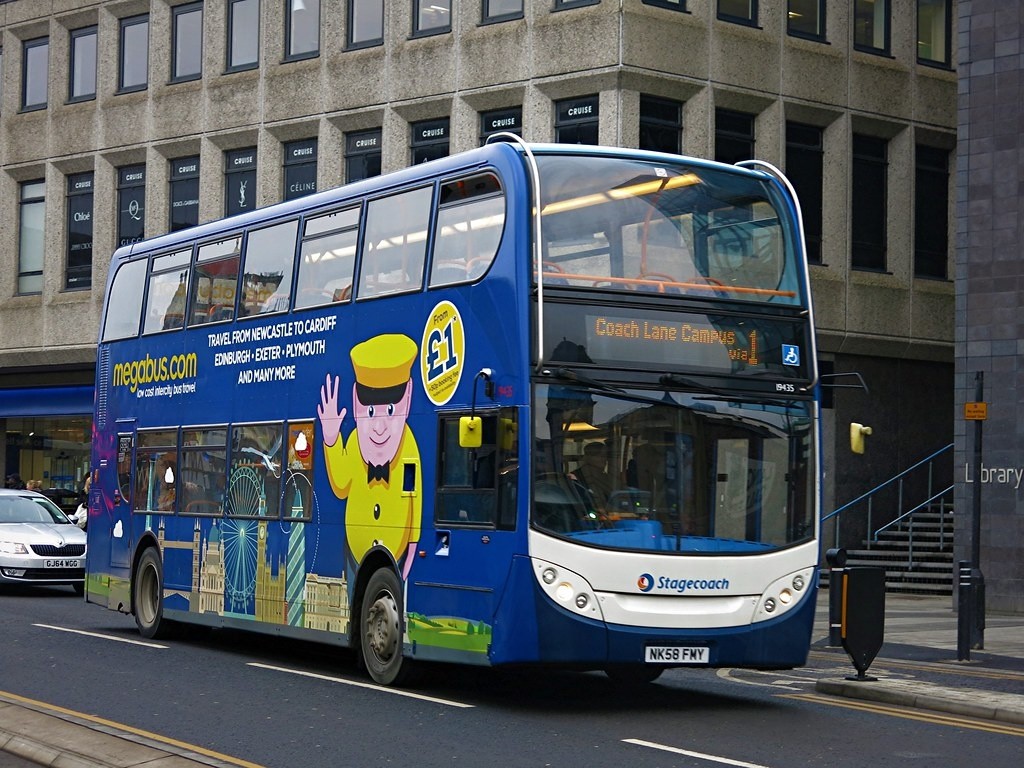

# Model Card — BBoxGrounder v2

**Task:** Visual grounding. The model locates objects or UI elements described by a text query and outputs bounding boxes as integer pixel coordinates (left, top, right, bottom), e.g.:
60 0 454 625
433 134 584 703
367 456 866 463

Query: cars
0 486 88 592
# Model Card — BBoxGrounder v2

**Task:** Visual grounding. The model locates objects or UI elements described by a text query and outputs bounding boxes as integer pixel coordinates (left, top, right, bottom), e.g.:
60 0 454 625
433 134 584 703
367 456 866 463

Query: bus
86 132 874 692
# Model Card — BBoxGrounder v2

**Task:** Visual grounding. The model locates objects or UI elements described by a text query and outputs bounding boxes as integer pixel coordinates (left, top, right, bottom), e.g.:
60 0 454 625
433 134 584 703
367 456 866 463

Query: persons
1 431 714 559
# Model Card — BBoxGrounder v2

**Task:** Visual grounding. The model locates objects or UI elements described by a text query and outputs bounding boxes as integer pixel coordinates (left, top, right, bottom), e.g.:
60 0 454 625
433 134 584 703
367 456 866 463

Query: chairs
151 246 739 318
